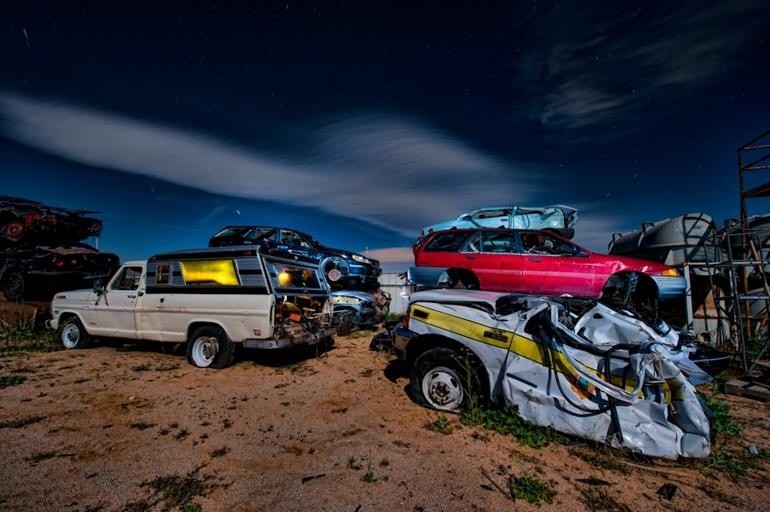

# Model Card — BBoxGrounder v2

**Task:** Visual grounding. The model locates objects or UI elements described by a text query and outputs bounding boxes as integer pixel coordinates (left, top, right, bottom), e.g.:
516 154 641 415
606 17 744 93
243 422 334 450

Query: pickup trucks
49 242 336 370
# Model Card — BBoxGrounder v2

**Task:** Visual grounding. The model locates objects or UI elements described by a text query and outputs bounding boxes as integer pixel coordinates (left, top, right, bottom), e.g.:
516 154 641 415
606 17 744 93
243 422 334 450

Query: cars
1 194 121 303
206 222 393 334
